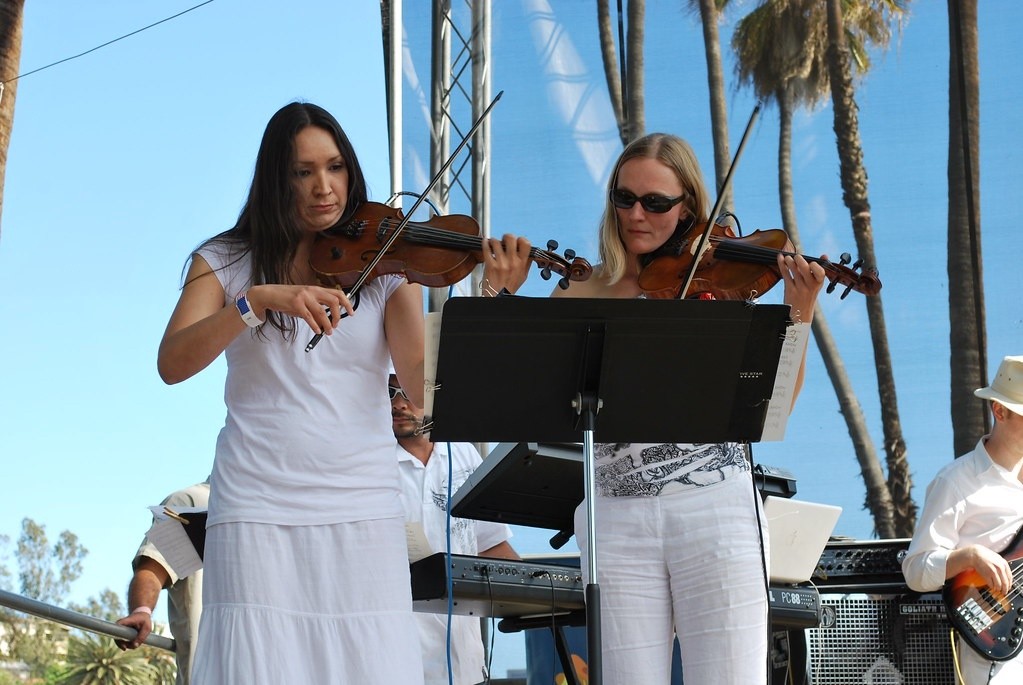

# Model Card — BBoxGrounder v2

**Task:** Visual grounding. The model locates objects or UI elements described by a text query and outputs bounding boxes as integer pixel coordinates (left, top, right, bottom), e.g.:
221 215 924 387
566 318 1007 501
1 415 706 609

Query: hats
974 356 1023 416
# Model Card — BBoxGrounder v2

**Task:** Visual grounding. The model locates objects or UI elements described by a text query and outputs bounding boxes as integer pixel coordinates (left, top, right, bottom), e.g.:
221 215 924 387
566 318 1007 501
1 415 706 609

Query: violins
637 221 883 301
309 201 593 291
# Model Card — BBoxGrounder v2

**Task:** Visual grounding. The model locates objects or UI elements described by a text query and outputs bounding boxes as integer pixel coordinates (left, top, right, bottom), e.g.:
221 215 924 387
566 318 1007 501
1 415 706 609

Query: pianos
409 552 822 627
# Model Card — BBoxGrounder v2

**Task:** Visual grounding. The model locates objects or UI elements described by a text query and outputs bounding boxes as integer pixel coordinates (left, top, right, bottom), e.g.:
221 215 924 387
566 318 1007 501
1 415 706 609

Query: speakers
770 584 957 685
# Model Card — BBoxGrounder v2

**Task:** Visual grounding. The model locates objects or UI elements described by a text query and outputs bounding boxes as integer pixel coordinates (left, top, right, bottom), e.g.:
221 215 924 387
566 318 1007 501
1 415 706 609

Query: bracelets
132 606 151 615
234 291 266 327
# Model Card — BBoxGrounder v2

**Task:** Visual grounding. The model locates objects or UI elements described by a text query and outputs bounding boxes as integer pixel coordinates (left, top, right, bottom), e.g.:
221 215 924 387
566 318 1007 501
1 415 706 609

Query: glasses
609 188 686 213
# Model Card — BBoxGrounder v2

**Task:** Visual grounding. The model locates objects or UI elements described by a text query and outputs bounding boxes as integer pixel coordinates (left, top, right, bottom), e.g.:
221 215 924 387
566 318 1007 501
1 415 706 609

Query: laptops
763 496 842 584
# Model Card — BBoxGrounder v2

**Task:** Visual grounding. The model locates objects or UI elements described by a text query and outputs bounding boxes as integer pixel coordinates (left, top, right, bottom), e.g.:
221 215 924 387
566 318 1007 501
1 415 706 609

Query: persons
157 101 533 685
900 354 1023 685
386 360 524 685
548 134 827 685
116 472 210 685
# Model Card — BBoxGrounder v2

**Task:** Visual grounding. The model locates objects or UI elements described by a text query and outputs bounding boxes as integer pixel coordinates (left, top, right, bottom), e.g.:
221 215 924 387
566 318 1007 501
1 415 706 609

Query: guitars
941 523 1023 661
